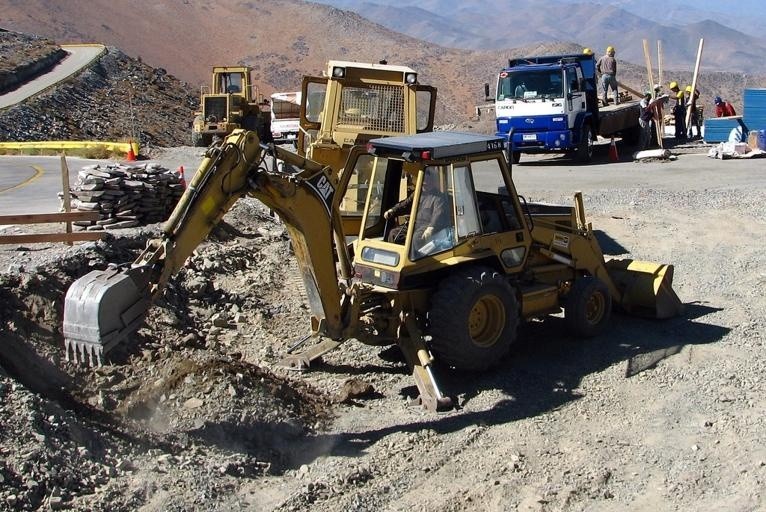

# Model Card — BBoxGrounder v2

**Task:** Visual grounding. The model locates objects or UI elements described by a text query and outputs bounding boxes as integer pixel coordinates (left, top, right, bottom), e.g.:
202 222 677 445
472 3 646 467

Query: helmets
715 97 721 104
686 86 691 92
670 82 678 89
607 46 614 52
583 48 591 55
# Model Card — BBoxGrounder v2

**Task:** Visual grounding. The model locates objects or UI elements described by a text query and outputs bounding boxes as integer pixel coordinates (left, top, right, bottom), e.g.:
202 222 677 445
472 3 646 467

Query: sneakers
604 101 621 106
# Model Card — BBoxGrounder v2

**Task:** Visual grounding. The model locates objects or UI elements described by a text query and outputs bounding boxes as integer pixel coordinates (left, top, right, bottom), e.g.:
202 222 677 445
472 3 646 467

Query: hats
654 84 662 89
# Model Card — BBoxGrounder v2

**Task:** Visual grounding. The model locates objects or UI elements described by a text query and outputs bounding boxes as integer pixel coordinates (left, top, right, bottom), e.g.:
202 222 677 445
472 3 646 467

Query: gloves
422 227 434 240
383 209 393 219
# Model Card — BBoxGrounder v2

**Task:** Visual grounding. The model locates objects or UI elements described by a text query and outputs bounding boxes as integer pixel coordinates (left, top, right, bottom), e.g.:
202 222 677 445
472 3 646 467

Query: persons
651 86 668 144
386 86 403 121
639 92 662 149
684 86 703 141
585 48 598 87
667 82 688 144
384 170 449 260
595 48 621 107
714 97 737 119
